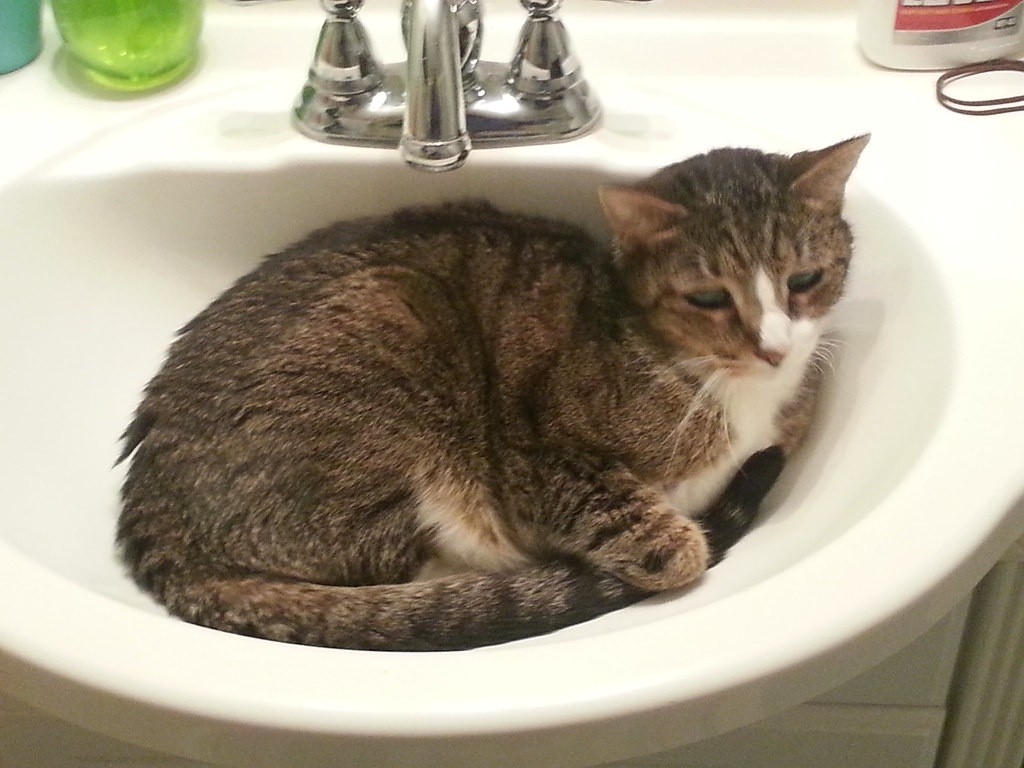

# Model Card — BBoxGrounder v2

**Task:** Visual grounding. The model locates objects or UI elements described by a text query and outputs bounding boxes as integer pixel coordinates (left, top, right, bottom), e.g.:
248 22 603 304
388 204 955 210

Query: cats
120 134 872 651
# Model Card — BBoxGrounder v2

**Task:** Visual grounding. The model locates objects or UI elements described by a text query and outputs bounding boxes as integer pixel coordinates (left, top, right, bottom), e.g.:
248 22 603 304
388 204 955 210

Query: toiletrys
49 1 206 96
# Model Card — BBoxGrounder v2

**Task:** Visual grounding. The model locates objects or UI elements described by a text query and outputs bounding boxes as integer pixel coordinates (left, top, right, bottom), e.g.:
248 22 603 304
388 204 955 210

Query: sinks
0 0 1021 765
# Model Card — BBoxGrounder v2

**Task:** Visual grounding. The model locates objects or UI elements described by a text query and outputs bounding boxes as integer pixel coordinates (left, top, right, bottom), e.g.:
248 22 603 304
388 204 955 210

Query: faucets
396 1 485 176
